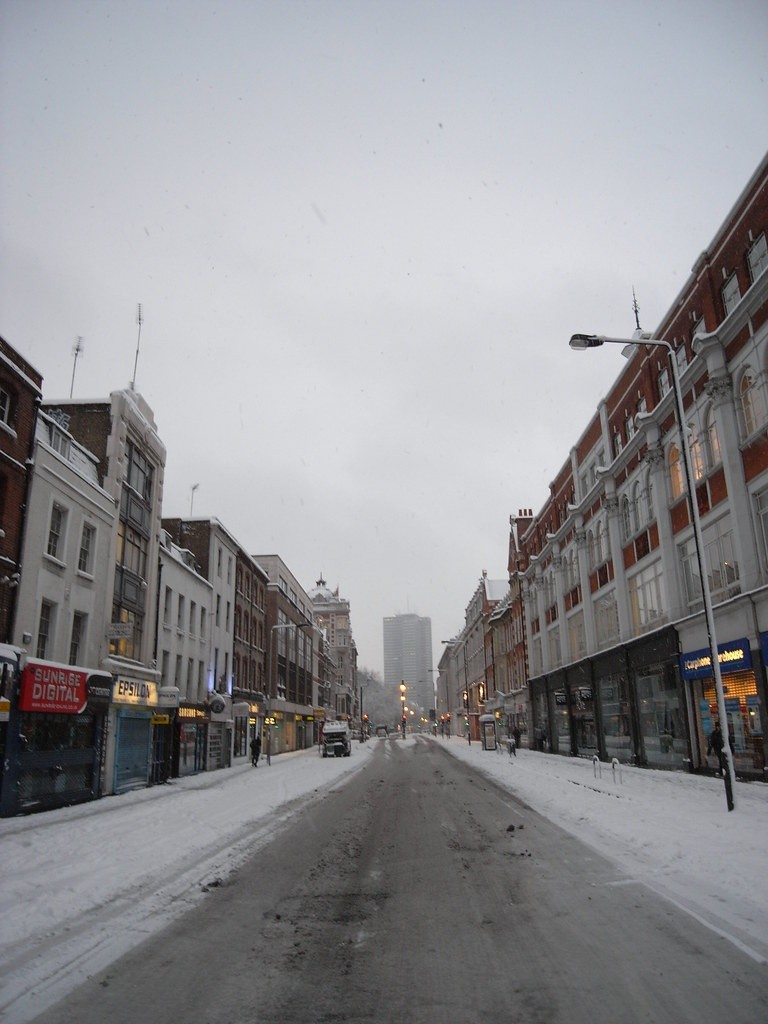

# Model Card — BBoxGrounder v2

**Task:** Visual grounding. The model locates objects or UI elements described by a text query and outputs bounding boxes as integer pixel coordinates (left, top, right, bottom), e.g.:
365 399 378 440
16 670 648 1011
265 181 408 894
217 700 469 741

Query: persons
250 735 262 768
512 726 522 748
707 721 734 778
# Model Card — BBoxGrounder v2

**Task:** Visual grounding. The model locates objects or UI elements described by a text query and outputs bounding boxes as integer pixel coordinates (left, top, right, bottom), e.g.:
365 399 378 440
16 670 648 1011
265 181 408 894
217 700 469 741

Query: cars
353 730 370 739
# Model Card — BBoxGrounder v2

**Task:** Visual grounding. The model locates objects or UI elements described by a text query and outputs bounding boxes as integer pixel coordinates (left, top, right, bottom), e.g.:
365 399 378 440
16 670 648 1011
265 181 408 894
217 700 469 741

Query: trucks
322 720 351 758
376 726 387 737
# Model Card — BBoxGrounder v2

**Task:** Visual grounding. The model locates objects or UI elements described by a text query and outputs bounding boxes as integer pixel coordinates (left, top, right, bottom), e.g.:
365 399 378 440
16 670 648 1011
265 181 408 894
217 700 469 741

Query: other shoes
254 763 257 767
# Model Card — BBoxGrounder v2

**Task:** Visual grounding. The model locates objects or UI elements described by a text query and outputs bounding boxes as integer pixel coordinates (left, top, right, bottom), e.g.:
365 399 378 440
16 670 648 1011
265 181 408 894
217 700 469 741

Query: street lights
568 333 739 812
441 640 471 745
400 680 406 739
267 623 310 765
428 669 450 739
419 680 437 737
